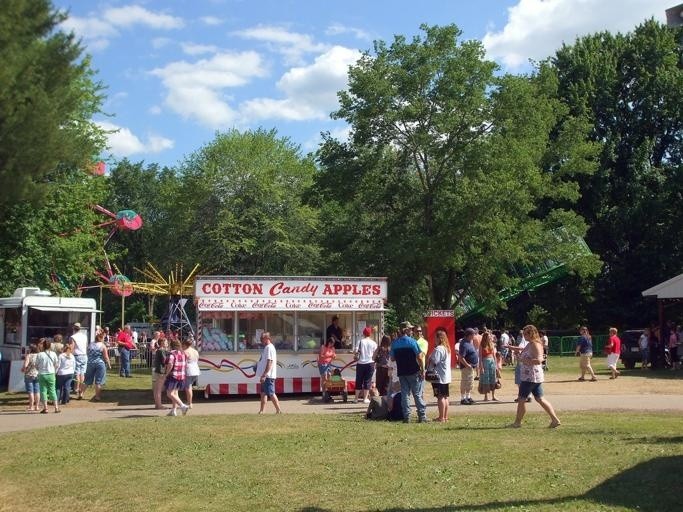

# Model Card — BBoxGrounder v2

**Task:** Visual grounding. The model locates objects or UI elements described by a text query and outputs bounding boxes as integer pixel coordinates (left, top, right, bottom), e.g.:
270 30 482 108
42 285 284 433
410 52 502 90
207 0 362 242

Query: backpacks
387 393 405 421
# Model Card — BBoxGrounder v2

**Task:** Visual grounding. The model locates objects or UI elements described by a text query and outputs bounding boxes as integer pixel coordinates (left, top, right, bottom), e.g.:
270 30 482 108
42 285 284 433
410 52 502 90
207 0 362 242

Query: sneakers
403 418 409 423
181 404 189 415
167 411 178 416
419 418 431 424
461 398 475 404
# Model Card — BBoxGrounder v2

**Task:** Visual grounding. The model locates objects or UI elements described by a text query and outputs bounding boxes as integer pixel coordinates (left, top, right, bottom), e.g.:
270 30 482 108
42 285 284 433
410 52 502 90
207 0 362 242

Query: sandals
40 409 48 414
434 418 448 422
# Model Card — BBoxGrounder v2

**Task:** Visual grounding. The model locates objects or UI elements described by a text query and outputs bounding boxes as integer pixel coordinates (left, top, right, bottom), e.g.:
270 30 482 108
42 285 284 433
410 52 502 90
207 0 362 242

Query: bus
126 322 159 341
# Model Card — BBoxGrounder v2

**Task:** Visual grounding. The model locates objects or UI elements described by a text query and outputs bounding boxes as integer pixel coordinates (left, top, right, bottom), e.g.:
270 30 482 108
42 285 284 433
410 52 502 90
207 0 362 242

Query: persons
388 321 428 424
427 330 452 423
477 332 502 402
312 313 430 407
504 325 563 430
254 332 281 414
19 319 201 417
458 327 549 373
458 328 479 405
604 328 623 380
639 320 678 372
573 326 597 382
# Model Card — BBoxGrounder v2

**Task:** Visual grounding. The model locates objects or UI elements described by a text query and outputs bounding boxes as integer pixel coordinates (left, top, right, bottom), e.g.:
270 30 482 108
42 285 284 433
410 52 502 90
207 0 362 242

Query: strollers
323 367 348 403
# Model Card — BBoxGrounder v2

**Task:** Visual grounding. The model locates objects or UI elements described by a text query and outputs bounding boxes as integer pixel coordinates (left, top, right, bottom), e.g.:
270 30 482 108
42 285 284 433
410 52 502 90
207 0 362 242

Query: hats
363 327 372 335
464 328 476 337
400 321 414 333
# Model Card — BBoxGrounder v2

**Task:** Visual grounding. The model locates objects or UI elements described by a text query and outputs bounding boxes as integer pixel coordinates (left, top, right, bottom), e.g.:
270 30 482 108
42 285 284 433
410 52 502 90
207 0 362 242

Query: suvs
621 329 670 369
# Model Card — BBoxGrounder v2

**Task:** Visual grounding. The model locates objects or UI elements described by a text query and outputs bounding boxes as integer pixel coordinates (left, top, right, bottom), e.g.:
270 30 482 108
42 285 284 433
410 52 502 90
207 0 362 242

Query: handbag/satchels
495 382 501 389
425 371 440 380
